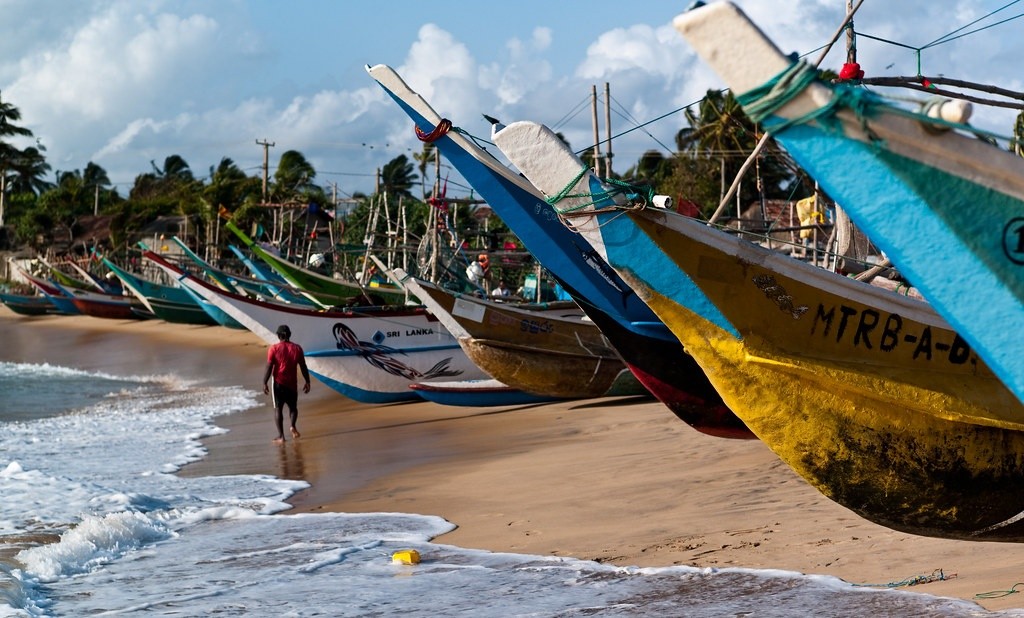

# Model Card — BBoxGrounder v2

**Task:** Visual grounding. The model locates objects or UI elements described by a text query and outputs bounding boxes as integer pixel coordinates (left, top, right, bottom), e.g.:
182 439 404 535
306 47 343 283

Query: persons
262 325 310 442
105 271 122 289
492 280 511 303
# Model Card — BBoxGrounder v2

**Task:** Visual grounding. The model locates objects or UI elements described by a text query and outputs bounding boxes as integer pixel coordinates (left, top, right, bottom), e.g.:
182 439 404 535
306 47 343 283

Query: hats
277 325 291 334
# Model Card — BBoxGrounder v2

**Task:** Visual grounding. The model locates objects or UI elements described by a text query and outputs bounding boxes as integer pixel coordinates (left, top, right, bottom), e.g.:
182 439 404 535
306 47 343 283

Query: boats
0 0 1023 549
